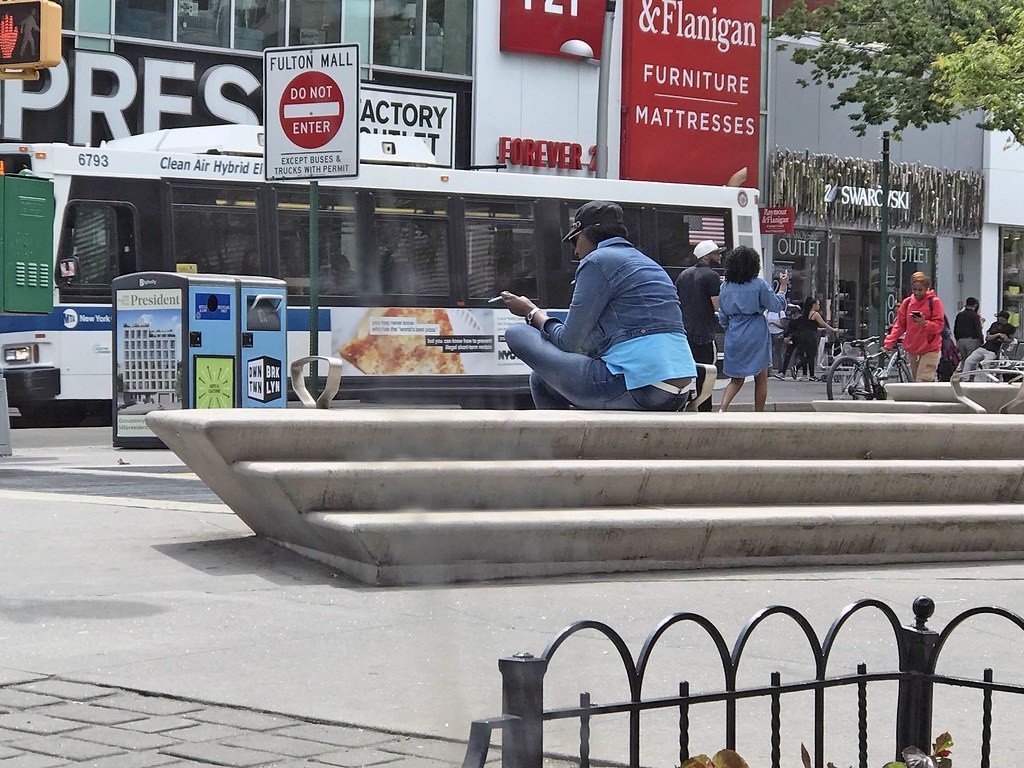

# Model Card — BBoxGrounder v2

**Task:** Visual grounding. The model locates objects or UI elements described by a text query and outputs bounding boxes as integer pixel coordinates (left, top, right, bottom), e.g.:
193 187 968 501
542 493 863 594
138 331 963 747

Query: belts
651 379 693 395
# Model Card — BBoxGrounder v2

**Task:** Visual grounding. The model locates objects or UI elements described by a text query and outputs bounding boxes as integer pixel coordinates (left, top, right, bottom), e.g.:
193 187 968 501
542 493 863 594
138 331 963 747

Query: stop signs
279 71 344 150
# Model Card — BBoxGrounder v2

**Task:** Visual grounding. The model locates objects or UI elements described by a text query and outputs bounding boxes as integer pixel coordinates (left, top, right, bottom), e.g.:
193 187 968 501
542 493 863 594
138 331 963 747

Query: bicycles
827 335 914 401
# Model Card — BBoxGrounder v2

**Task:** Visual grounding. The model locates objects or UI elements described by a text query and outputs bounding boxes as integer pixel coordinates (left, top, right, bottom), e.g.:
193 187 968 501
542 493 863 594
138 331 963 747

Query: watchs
527 308 541 325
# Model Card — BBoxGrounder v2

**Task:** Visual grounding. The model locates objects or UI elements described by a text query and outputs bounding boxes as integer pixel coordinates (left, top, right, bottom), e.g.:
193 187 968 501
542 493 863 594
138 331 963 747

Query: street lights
879 130 891 366
560 39 611 178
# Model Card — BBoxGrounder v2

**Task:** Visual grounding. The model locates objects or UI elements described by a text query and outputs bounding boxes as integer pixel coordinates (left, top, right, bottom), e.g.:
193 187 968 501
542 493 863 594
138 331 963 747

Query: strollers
816 332 847 383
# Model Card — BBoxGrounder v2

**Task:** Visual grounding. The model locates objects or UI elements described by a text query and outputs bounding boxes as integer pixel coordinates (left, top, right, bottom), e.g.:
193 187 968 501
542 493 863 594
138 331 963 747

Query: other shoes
774 372 786 381
791 366 798 380
799 375 808 381
809 376 819 382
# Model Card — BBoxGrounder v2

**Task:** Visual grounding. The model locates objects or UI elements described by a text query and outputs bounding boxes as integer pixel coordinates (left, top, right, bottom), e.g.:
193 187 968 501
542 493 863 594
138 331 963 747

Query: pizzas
338 308 466 375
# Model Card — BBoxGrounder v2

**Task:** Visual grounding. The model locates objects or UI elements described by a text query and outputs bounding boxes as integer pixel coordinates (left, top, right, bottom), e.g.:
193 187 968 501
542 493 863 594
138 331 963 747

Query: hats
994 311 1010 320
787 302 801 310
693 240 727 259
562 200 623 243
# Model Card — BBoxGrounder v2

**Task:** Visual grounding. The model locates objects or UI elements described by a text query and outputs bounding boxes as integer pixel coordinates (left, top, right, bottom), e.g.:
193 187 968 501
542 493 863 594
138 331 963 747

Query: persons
718 245 789 411
763 296 838 383
1002 267 1023 294
954 296 1016 383
676 239 721 413
884 272 944 382
500 200 699 411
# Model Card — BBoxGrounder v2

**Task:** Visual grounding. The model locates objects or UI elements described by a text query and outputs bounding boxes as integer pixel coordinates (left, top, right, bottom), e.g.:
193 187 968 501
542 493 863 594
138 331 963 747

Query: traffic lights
0 0 62 80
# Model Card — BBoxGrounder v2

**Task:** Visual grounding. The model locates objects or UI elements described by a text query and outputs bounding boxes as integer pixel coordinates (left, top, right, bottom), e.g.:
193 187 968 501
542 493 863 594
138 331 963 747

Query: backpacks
905 297 952 350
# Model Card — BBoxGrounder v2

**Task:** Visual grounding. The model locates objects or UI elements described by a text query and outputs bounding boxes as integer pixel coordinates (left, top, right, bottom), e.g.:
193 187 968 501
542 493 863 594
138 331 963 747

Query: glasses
568 223 600 246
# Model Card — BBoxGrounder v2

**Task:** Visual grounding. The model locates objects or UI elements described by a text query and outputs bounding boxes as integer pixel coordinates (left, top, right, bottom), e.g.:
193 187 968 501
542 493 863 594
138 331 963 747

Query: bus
0 125 762 427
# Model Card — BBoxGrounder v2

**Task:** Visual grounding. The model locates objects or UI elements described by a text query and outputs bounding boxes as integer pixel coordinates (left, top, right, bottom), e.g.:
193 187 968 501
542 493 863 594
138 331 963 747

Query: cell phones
911 311 921 322
